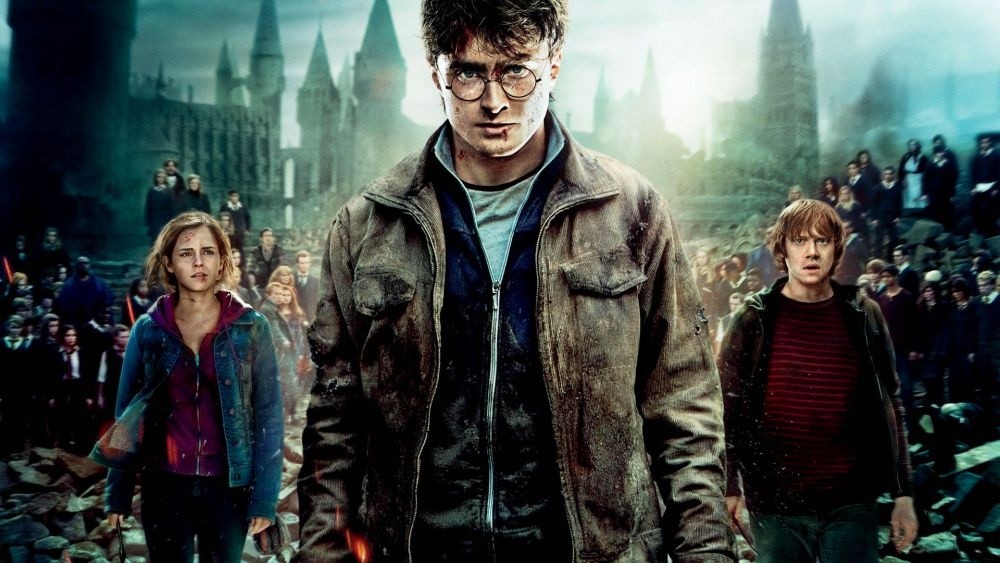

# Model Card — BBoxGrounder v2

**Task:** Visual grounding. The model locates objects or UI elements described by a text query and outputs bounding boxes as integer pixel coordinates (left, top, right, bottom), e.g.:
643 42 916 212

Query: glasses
435 60 548 101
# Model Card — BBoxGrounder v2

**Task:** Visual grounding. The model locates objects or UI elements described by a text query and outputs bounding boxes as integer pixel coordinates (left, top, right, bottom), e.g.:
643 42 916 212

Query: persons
300 0 739 562
0 134 1000 563
